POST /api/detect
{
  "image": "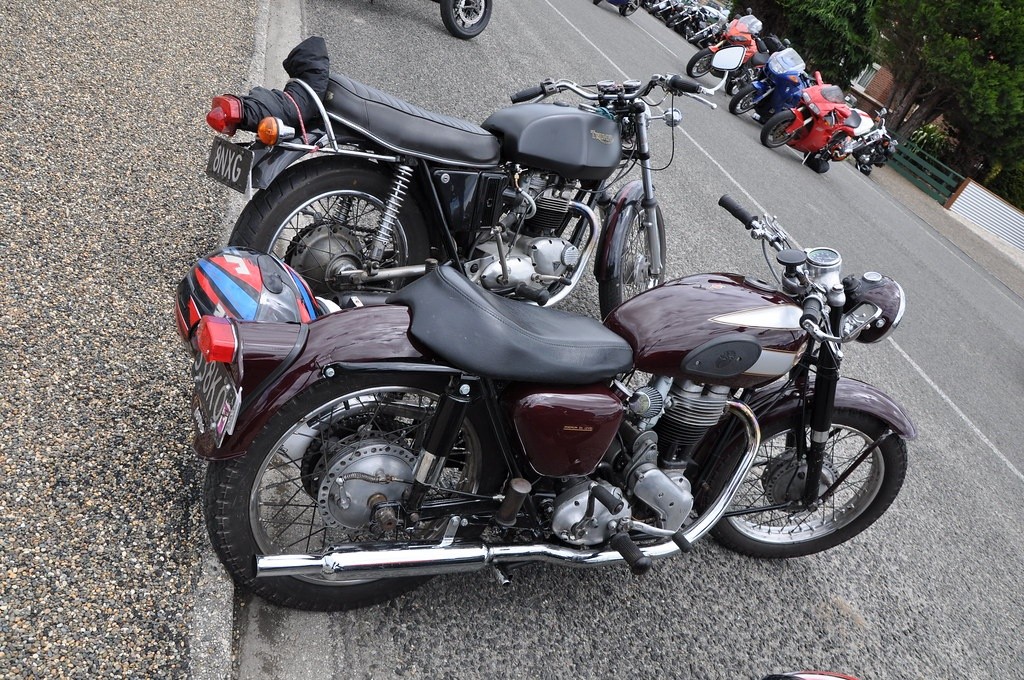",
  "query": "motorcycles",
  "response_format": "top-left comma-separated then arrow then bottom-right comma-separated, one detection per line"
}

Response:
760,71 -> 876,174
175,195 -> 919,608
729,33 -> 815,126
430,0 -> 493,40
686,7 -> 770,81
206,70 -> 718,320
851,107 -> 899,176
593,0 -> 731,44
